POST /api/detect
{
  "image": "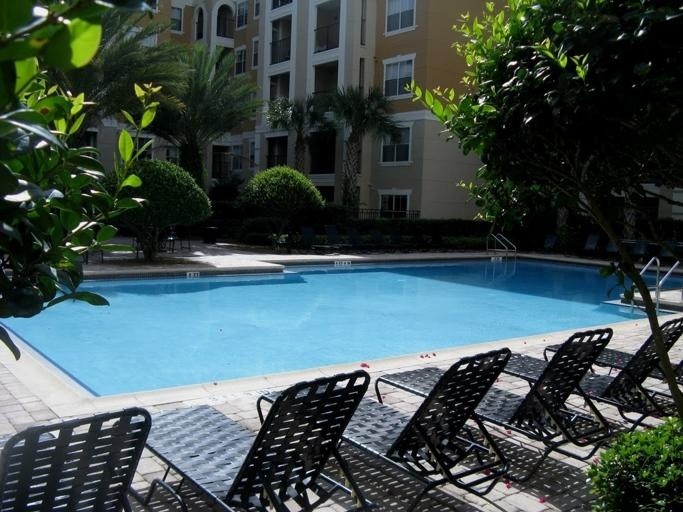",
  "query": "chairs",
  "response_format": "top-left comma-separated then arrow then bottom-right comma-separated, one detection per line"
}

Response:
82,246 -> 105,265
131,234 -> 191,255
310,225 -> 401,253
